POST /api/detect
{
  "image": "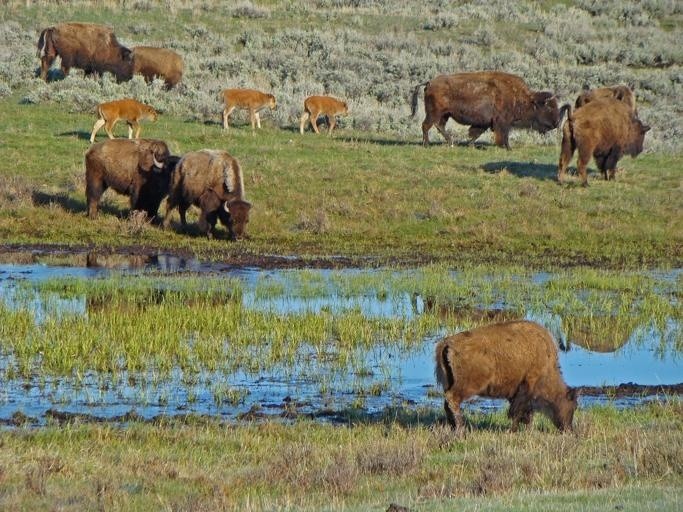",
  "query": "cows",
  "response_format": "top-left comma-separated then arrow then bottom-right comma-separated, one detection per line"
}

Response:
37,22 -> 186,92
85,137 -> 181,225
162,150 -> 254,242
299,96 -> 349,138
434,320 -> 585,437
558,71 -> 652,188
408,70 -> 560,153
88,98 -> 158,144
220,88 -> 276,130
